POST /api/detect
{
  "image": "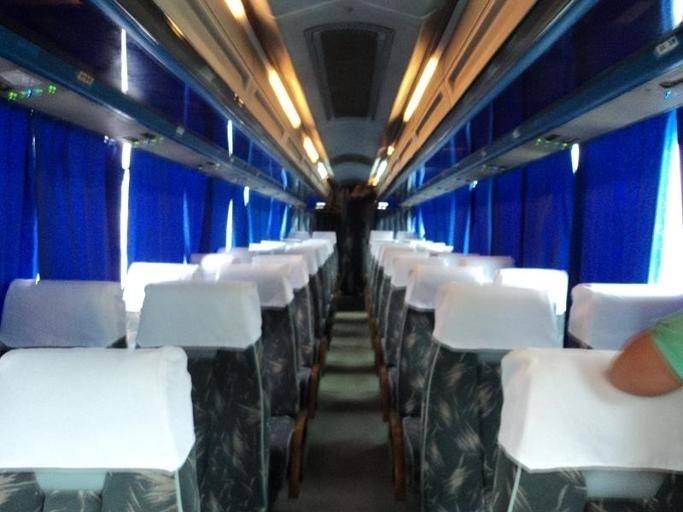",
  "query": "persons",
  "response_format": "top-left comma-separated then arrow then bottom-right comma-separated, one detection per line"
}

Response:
610,308 -> 683,397
342,182 -> 376,296
